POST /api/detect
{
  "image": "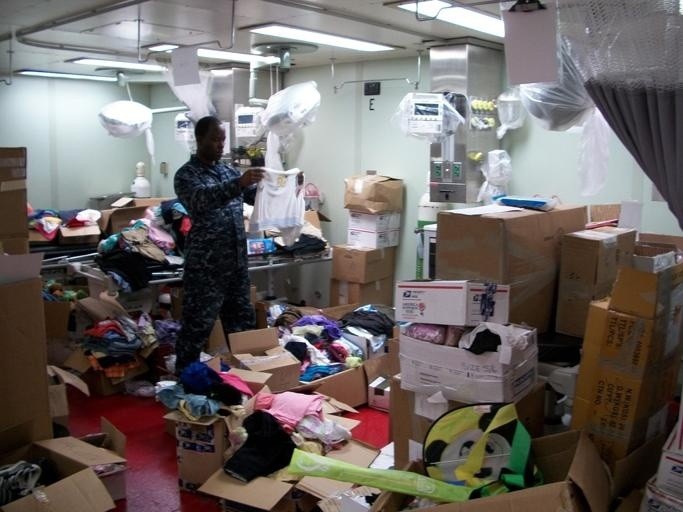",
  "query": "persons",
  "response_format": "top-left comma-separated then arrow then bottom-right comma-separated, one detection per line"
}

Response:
172,115 -> 304,381
